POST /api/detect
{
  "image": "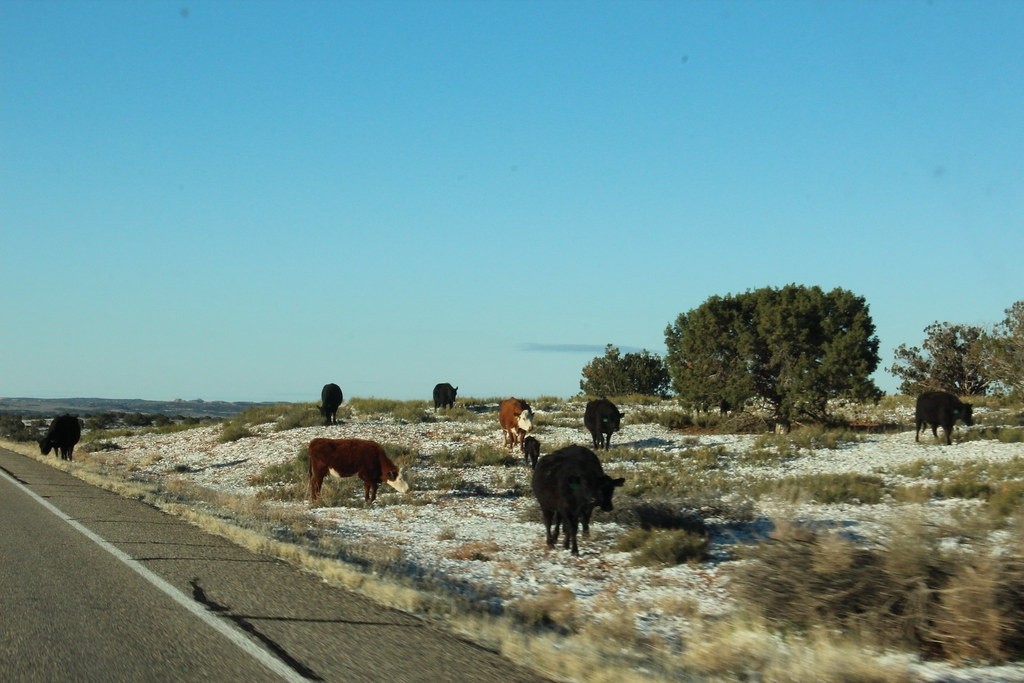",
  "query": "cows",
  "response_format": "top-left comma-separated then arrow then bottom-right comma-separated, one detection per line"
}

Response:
914,390 -> 975,446
584,399 -> 625,452
316,382 -> 343,426
36,413 -> 82,461
531,443 -> 626,557
432,382 -> 460,414
498,396 -> 534,453
524,435 -> 541,471
307,437 -> 410,508
